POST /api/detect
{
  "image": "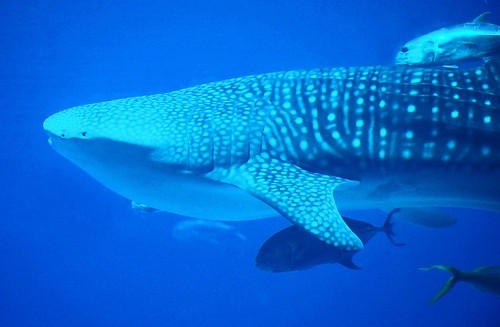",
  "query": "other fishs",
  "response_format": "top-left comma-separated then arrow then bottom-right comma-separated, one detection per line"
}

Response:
396,12 -> 500,69
416,263 -> 500,304
255,208 -> 408,273
172,220 -> 247,247
